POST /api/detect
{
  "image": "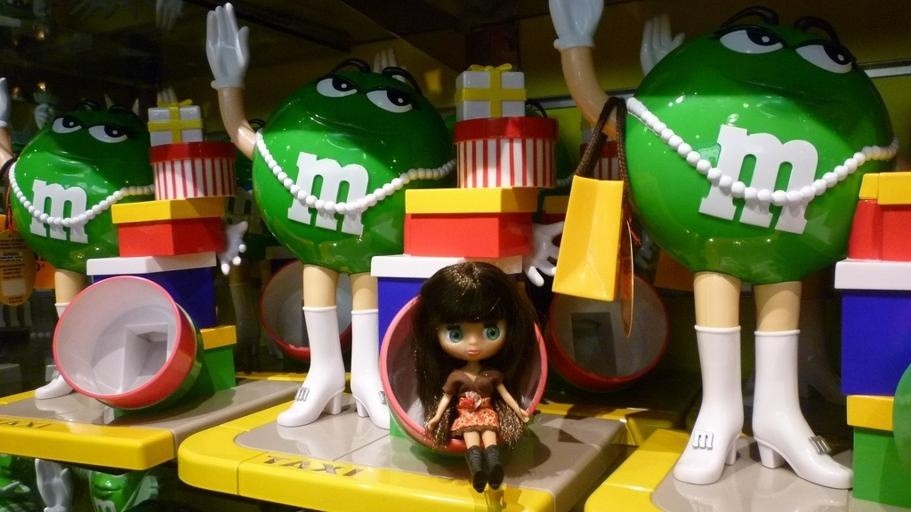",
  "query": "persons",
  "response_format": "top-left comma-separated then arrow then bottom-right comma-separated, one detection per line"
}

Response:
415,261 -> 540,496
